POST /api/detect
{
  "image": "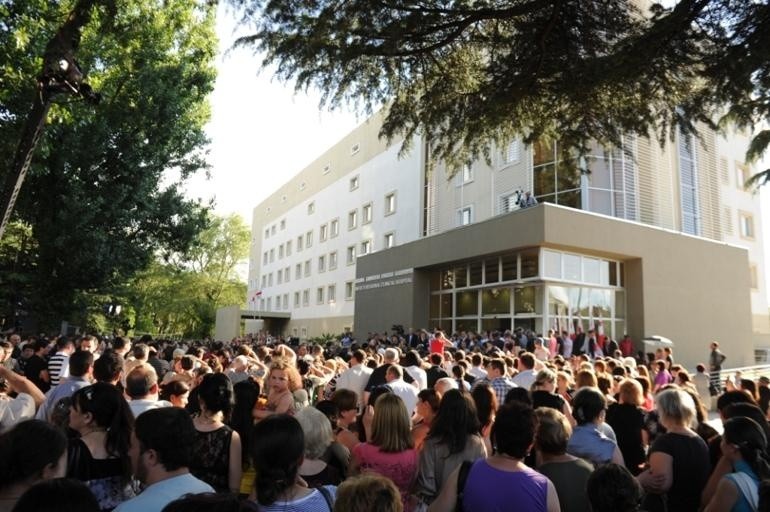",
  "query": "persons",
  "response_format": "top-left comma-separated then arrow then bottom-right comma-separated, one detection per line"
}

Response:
523,191 -> 538,208
